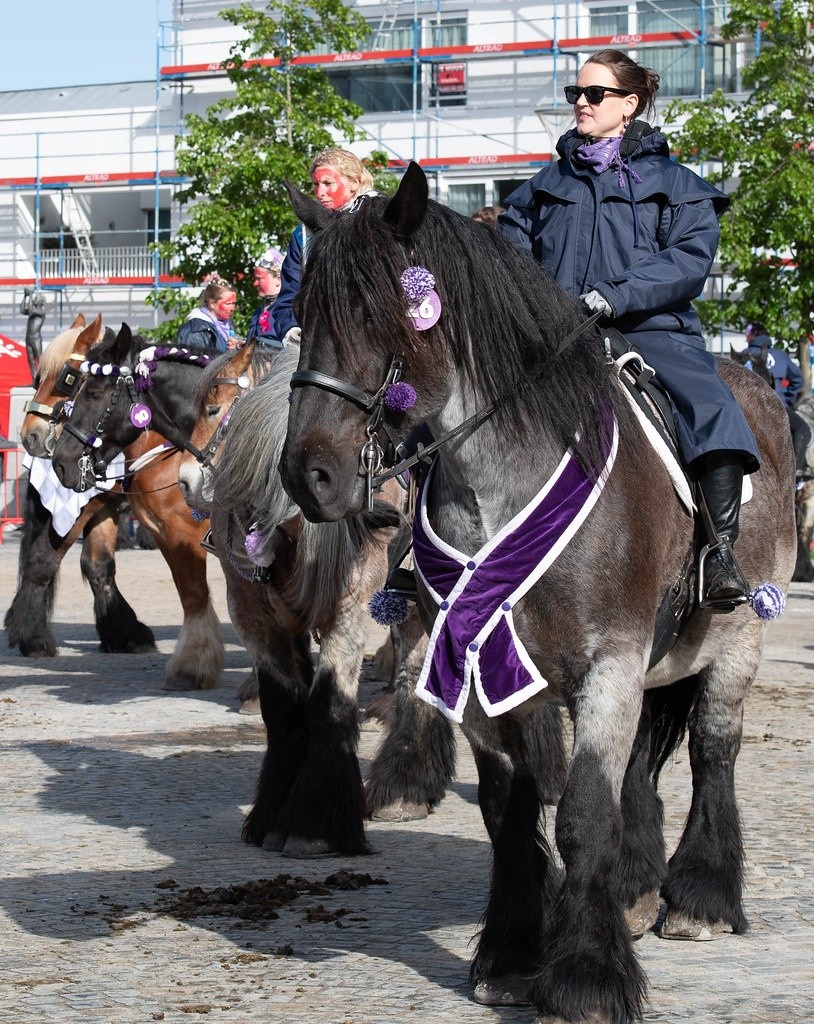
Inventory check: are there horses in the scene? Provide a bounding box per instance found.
[727,343,814,583]
[4,313,397,715]
[276,157,796,1023]
[209,336,456,857]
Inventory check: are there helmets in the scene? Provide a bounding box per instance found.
[745,323,770,341]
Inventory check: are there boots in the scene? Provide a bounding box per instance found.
[794,431,814,478]
[702,449,748,613]
[387,568,418,591]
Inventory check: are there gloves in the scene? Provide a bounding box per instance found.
[579,289,612,318]
[282,326,302,348]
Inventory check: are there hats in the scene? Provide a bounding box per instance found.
[254,246,286,272]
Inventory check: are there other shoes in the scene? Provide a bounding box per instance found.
[262,547,293,575]
[209,534,215,547]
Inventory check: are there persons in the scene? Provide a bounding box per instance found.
[175,279,241,352]
[739,323,814,483]
[498,48,761,614]
[471,206,505,227]
[246,245,286,341]
[272,149,373,349]
[20,293,47,383]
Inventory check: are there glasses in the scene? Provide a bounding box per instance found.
[564,85,633,105]
